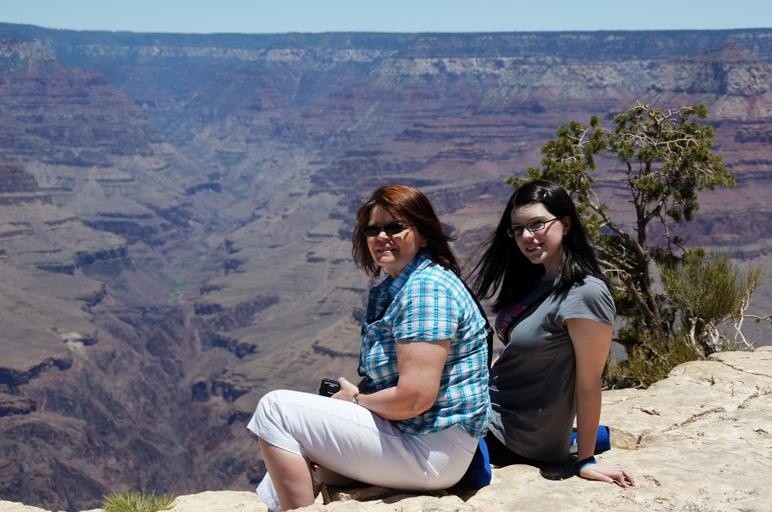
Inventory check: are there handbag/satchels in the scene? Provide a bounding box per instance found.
[570,424,610,455]
[455,436,492,488]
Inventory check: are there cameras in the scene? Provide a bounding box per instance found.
[320,379,341,397]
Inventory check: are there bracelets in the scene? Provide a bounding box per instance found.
[576,456,595,476]
[351,392,359,404]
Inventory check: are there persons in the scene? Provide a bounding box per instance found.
[464,177,638,490]
[245,184,492,511]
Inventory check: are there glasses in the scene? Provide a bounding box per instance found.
[506,217,560,238]
[363,220,417,237]
